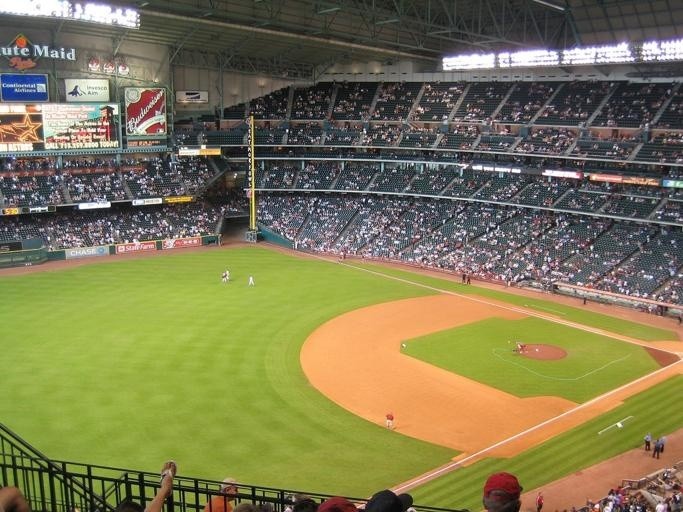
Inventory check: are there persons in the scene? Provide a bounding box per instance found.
[146,461,176,512]
[205,478,238,511]
[587,465,683,512]
[651,438,662,459]
[251,80,683,312]
[222,269,230,283]
[176,122,211,145]
[536,492,544,512]
[236,503,273,512]
[1,156,249,250]
[293,489,413,512]
[660,436,664,452]
[118,501,143,512]
[0,486,29,512]
[643,431,650,450]
[483,471,523,512]
[248,275,254,285]
[385,411,394,430]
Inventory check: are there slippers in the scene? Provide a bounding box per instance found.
[159,459,176,498]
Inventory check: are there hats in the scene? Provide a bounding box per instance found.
[365,489,413,512]
[481,472,523,501]
[220,477,242,490]
[317,496,359,511]
